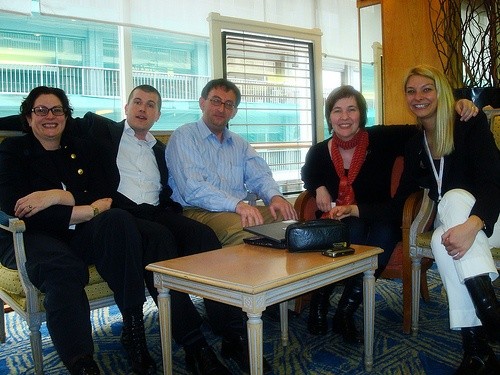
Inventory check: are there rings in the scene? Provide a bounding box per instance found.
[26,205,32,212]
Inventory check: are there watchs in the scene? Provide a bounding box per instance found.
[90,205,99,216]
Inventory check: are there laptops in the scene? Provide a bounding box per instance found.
[240,218,299,248]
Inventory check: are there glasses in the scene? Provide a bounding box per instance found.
[207,97,237,110]
[32,106,69,116]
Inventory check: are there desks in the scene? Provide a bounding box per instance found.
[146,244,385,375]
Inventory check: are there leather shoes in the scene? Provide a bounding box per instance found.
[185,338,233,375]
[222,332,274,375]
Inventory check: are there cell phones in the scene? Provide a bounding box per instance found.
[321,246,355,258]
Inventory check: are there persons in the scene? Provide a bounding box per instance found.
[165,79,299,322]
[0,84,280,375]
[301,85,478,345]
[328,65,500,375]
[0,86,157,375]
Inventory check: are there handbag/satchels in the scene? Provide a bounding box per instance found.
[285,219,351,252]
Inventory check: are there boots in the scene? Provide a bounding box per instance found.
[308,283,335,335]
[69,360,100,375]
[464,273,500,345]
[332,284,364,344]
[118,303,157,375]
[455,325,500,375]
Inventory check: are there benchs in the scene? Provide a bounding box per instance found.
[0,131,175,375]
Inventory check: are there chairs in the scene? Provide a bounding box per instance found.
[293,185,437,333]
[411,108,500,336]
